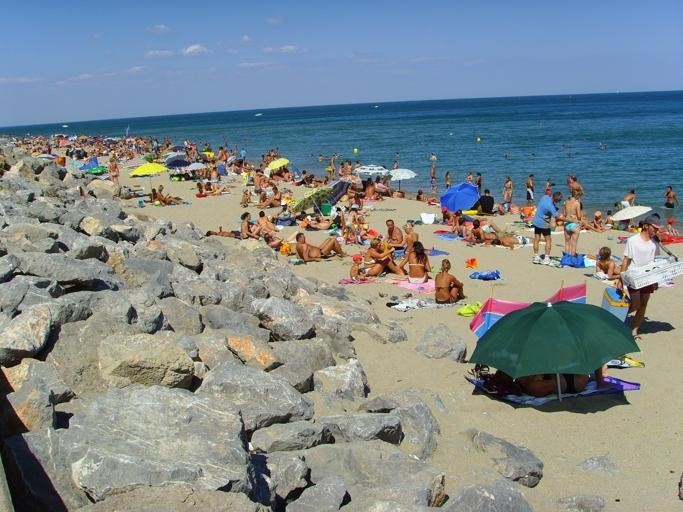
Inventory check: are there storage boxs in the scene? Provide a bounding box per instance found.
[600,286,631,323]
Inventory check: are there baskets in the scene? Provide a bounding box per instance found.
[620,256,682,290]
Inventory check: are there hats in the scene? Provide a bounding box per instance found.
[643,215,664,229]
